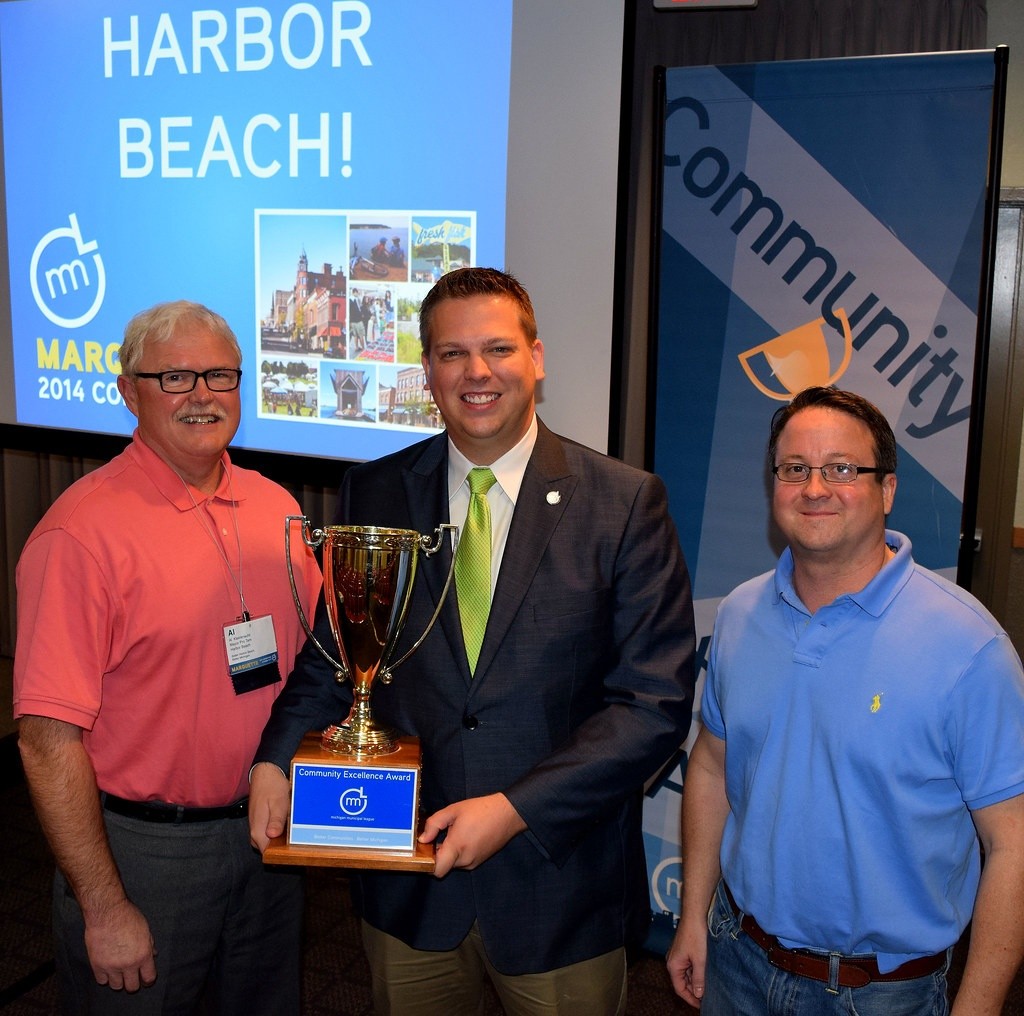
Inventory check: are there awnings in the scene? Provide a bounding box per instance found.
[392,409,420,414]
[379,409,386,413]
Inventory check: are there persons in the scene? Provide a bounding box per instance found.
[265,393,316,416]
[666,388,1024,1016]
[10,302,324,1016]
[350,287,394,352]
[249,266,695,1016]
[371,236,404,266]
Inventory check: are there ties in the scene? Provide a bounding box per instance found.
[453,467,497,677]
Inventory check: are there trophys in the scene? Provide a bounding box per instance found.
[264,516,459,872]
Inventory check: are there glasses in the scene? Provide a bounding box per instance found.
[133,367,244,395]
[772,461,893,484]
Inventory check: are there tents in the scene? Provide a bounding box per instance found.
[261,373,317,407]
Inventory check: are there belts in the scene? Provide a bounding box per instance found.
[103,792,250,825]
[723,880,946,989]
